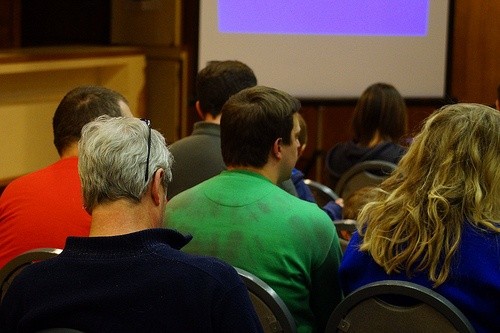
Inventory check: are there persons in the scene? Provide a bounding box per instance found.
[165,85,344,333]
[1,114,262,333]
[277,82,500,333]
[0,87,133,269]
[165,60,298,200]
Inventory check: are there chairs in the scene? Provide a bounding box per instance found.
[0,160,476,333]
